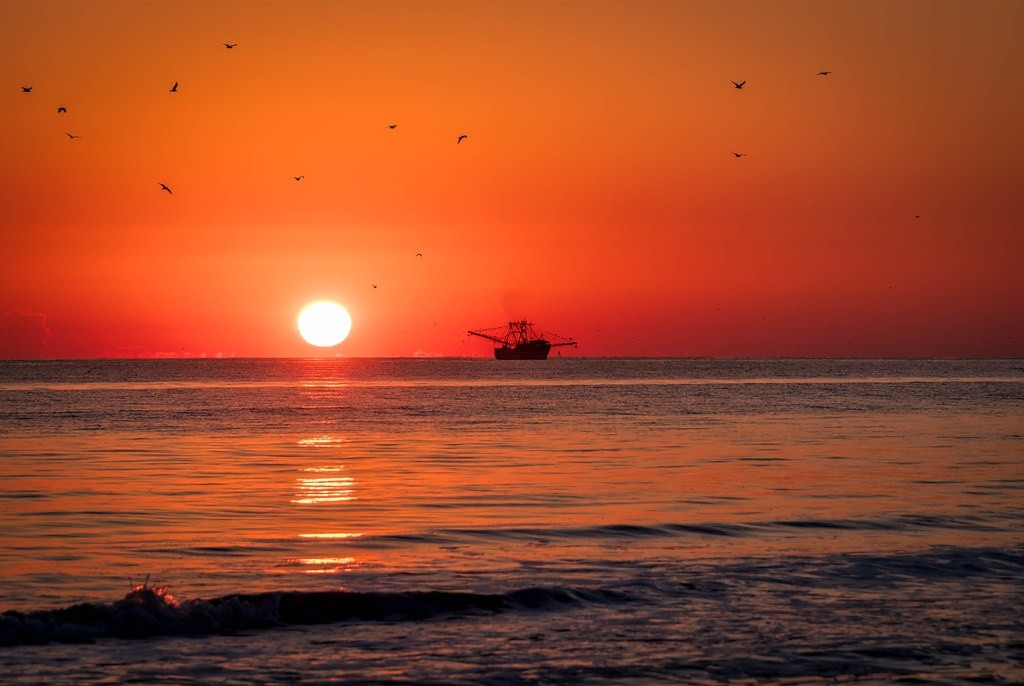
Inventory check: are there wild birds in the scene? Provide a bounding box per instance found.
[373,285,376,288]
[225,43,237,49]
[416,254,422,256]
[457,135,467,144]
[159,184,172,195]
[294,176,304,181]
[169,82,178,92]
[817,71,831,75]
[65,133,81,138]
[389,125,397,129]
[58,108,66,113]
[733,152,747,157]
[21,87,32,92]
[731,81,745,89]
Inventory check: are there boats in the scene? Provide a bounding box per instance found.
[467,317,580,361]
